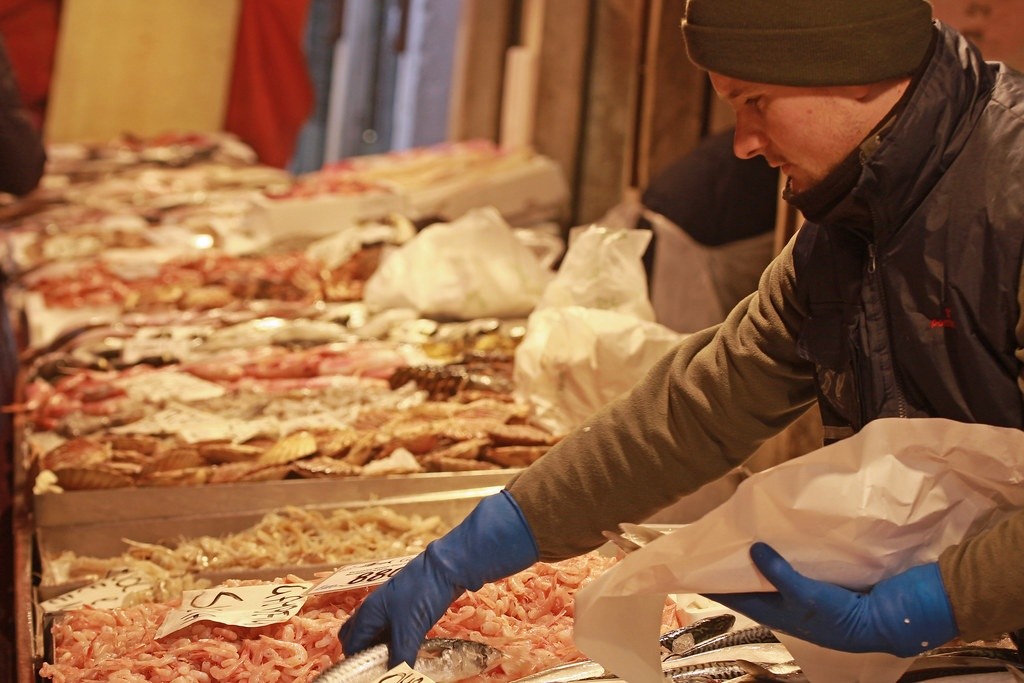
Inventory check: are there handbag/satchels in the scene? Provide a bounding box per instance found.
[366,207,564,320]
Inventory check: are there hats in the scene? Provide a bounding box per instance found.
[680,0,933,86]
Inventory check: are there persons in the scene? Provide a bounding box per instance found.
[629,125,780,333]
[337,0,1023,663]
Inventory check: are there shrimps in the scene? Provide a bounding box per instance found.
[39,507,684,682]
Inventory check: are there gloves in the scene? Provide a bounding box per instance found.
[704,543,960,658]
[337,490,540,671]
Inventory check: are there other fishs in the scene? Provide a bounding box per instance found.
[590,614,1024,683]
[0,129,571,493]
[308,637,504,683]
